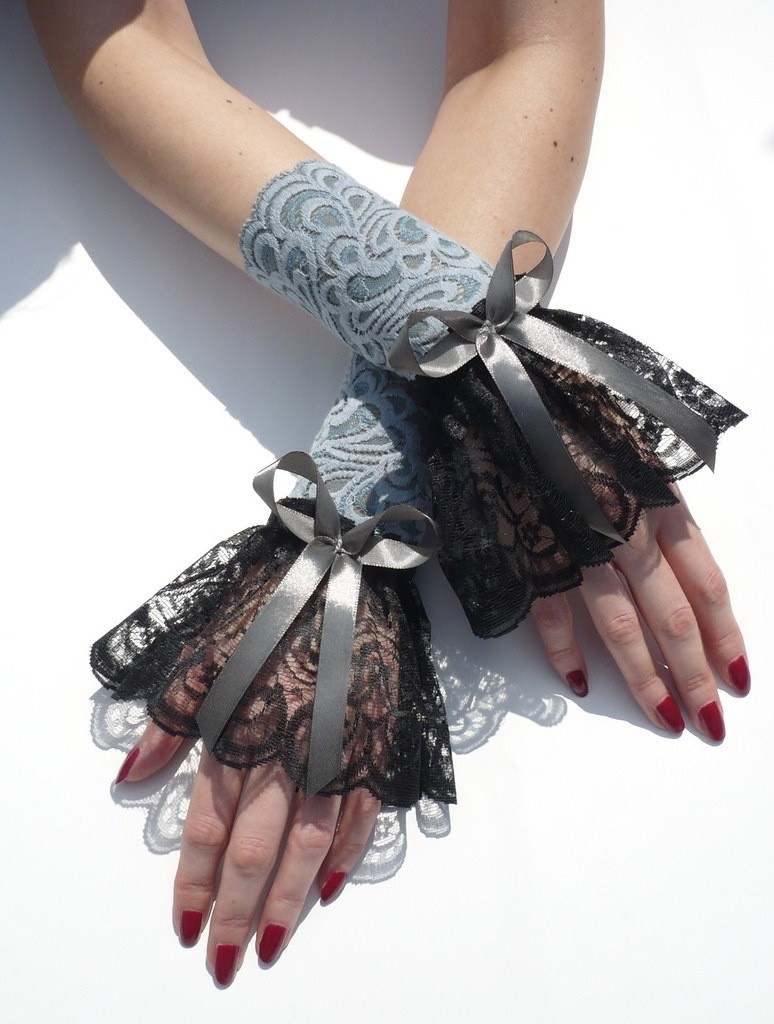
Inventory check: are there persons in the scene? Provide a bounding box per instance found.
[27,0,752,986]
